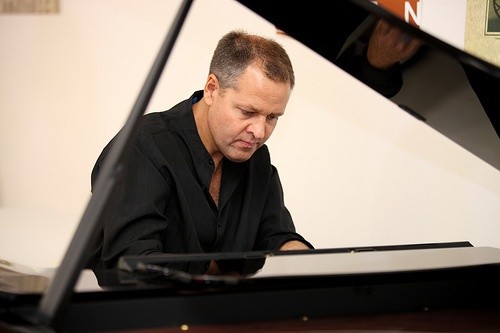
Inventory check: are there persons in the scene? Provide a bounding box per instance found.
[90,29,313,282]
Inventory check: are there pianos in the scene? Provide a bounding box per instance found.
[2,1,500,332]
